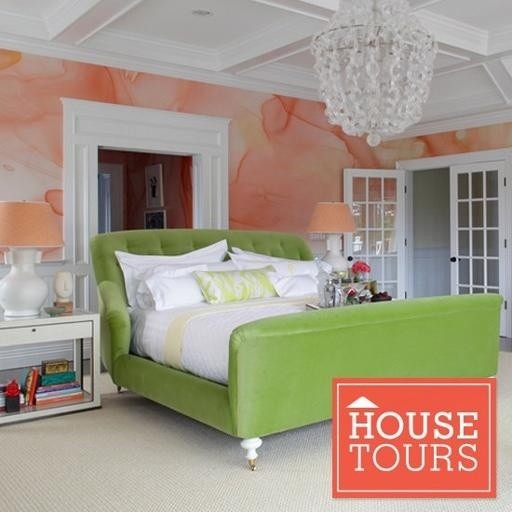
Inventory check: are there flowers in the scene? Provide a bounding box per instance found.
[351,263,371,274]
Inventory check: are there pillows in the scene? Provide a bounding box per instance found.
[112,239,331,312]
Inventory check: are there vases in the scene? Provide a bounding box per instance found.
[355,272,363,281]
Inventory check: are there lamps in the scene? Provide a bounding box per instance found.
[308,0,440,149]
[0,199,66,320]
[307,200,358,275]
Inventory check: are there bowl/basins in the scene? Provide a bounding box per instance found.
[43,307,65,318]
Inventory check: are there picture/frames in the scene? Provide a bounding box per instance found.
[144,163,165,209]
[144,210,167,230]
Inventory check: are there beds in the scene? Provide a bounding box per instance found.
[90,227,504,474]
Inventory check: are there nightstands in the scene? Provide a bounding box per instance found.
[1,309,102,425]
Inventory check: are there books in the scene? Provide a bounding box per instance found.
[0,359,85,412]
